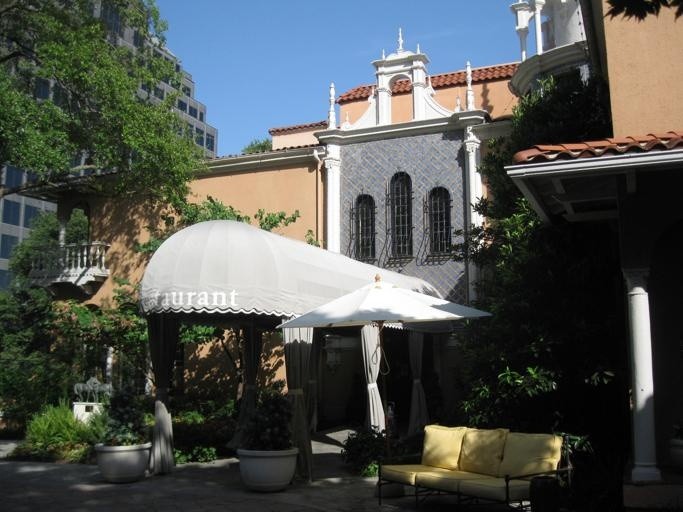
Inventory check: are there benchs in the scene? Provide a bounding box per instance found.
[377,424,574,512]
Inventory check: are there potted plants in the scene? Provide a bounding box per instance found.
[93,383,152,484]
[235,387,300,492]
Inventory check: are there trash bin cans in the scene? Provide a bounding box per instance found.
[73,401,104,424]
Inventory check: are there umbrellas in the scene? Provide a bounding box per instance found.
[274,273,494,464]
